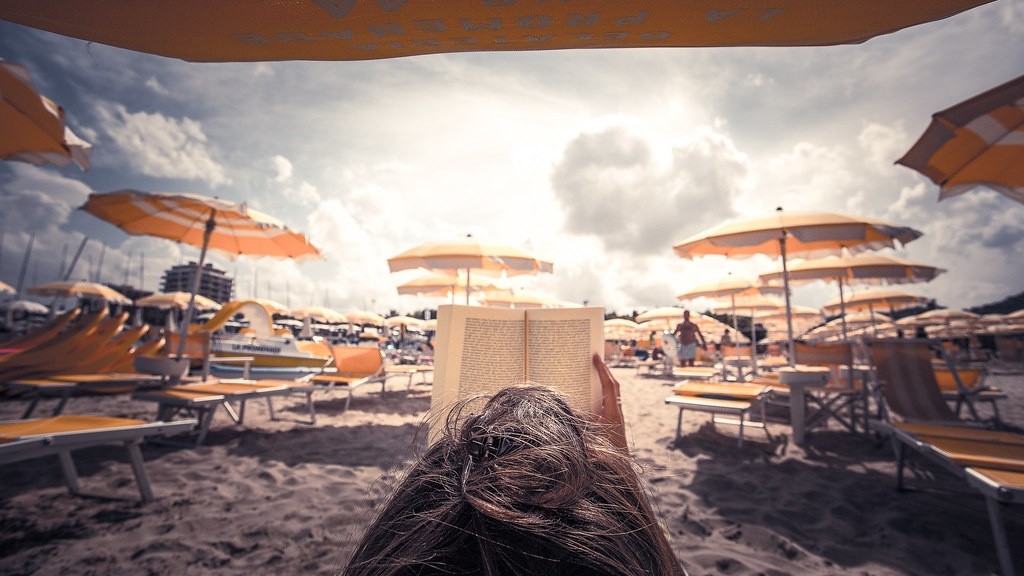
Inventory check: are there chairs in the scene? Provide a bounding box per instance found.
[0,302,1024,576]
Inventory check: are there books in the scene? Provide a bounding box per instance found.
[425,303,605,453]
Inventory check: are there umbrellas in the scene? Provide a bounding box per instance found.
[673,204,926,366]
[0,234,556,335]
[0,60,93,161]
[0,0,998,64]
[604,249,1024,347]
[79,188,326,359]
[893,75,1024,210]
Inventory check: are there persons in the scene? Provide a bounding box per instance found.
[333,353,692,576]
